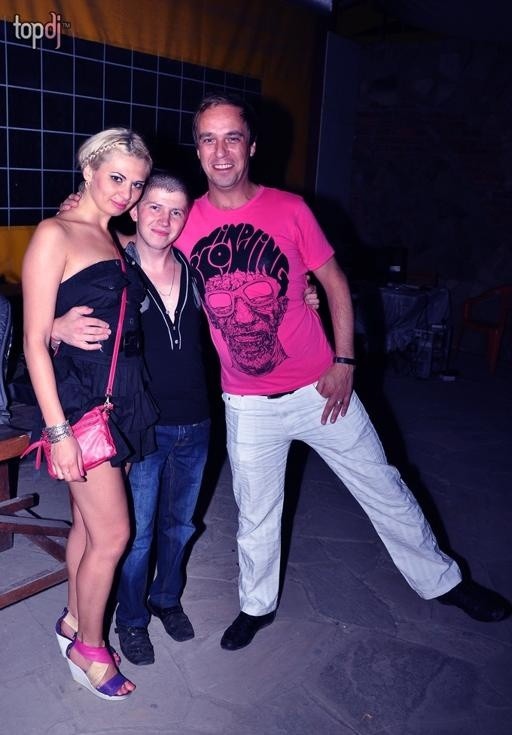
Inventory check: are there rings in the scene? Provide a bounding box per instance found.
[310,286,316,293]
[62,469,70,475]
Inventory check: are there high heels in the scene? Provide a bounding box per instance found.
[55,607,131,701]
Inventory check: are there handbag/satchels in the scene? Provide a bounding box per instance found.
[42,404,117,480]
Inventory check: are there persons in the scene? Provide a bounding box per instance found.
[17,128,163,700]
[46,171,324,663]
[53,95,512,652]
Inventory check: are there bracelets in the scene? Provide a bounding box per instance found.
[42,420,73,444]
[332,355,357,365]
[48,329,61,351]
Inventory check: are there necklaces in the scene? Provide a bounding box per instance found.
[143,259,175,298]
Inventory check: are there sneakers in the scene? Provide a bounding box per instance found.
[435,576,512,623]
[114,619,155,666]
[147,596,194,642]
[220,611,276,650]
